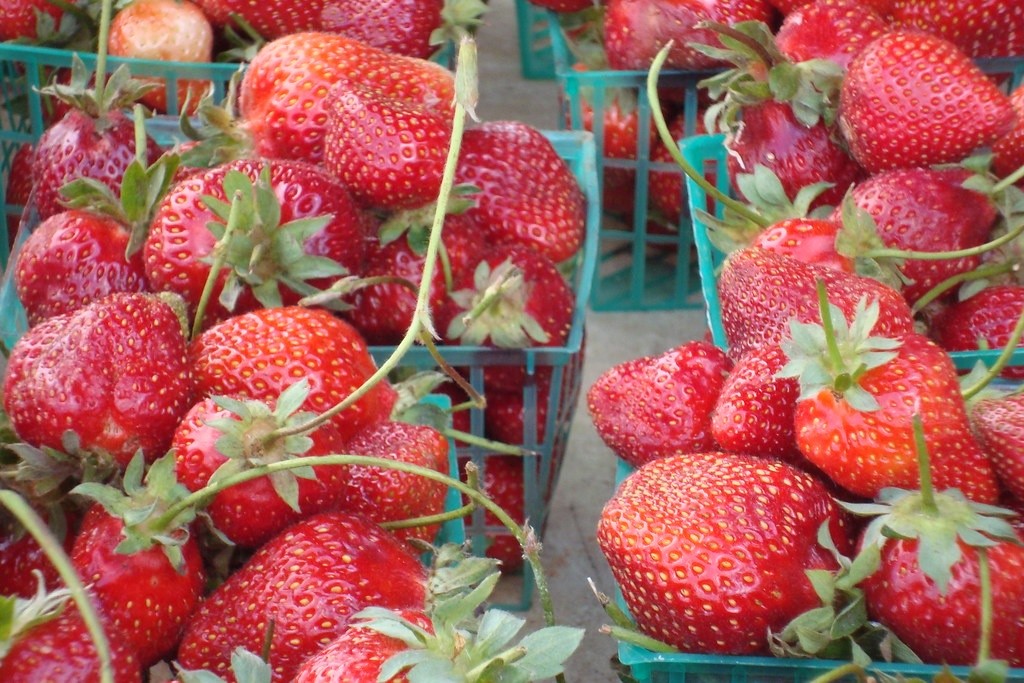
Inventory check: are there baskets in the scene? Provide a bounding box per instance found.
[614,378,1024,683]
[546,10,1024,311]
[0,0,489,268]
[0,109,601,613]
[0,393,466,683]
[516,0,606,78]
[678,134,1024,368]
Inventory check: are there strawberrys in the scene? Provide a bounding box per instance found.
[0,0,1024,682]
